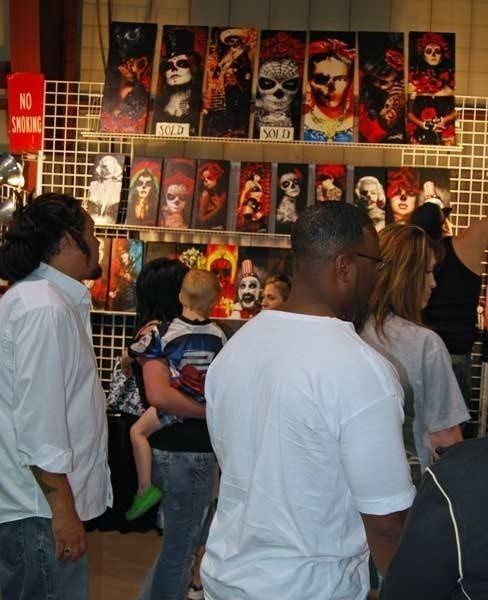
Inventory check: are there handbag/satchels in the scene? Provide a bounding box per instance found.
[106,323,166,418]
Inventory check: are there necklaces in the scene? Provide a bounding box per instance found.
[308,107,348,138]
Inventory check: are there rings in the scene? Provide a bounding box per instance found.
[63,547,71,554]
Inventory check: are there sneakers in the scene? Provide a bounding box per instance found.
[187,584,202,598]
[126,484,162,522]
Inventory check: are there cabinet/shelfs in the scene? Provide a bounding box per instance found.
[72,116,466,325]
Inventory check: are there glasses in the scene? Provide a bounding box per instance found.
[355,249,386,272]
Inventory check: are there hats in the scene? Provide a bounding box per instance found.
[117,239,128,254]
[410,202,452,235]
[423,181,439,200]
[240,260,258,278]
[129,162,159,192]
[221,29,249,47]
[318,165,344,178]
[164,159,193,193]
[115,32,149,65]
[164,29,198,56]
[256,167,264,176]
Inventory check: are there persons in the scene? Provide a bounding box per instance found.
[238,169,266,225]
[354,175,387,232]
[88,154,122,224]
[407,33,456,144]
[304,40,356,142]
[255,34,302,121]
[357,221,471,488]
[129,256,218,600]
[275,168,301,224]
[159,171,195,231]
[198,198,417,600]
[108,247,138,311]
[119,268,228,522]
[261,273,291,310]
[408,195,488,391]
[128,168,159,226]
[1,192,115,600]
[151,27,201,136]
[387,167,420,225]
[198,161,227,226]
[235,267,267,316]
[218,28,253,133]
